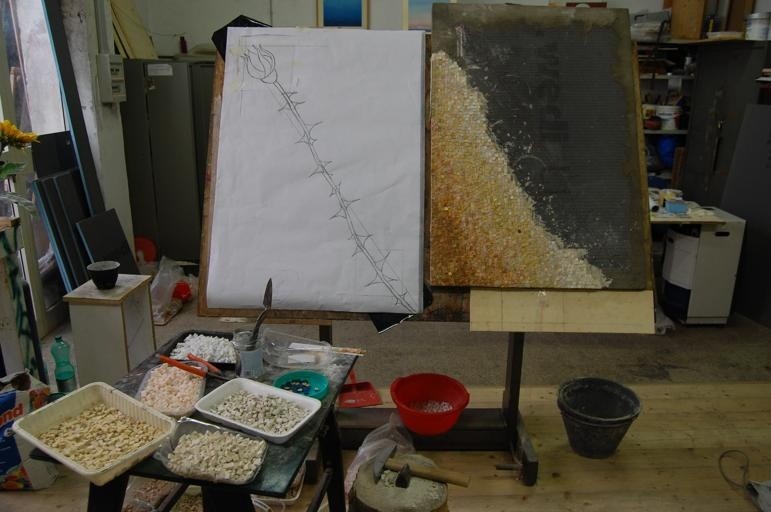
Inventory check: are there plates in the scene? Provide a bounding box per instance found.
[197,377,322,443]
[163,328,241,370]
[13,382,174,486]
[154,416,268,483]
[133,362,209,417]
[272,369,329,399]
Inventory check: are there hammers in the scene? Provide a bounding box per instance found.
[371,443,470,491]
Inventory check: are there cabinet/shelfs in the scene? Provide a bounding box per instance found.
[120,54,220,275]
[658,202,746,331]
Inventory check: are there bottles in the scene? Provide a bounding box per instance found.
[50,335,77,394]
[745,11,770,41]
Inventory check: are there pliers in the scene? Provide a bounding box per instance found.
[151,352,227,382]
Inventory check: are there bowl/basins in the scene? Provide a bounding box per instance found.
[559,377,642,459]
[88,260,120,290]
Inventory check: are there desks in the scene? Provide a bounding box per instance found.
[85,329,362,511]
[61,273,155,386]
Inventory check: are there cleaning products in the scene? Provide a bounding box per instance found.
[51,335,75,380]
[0,389,60,491]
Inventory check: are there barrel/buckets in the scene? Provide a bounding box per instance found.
[556,377,642,458]
